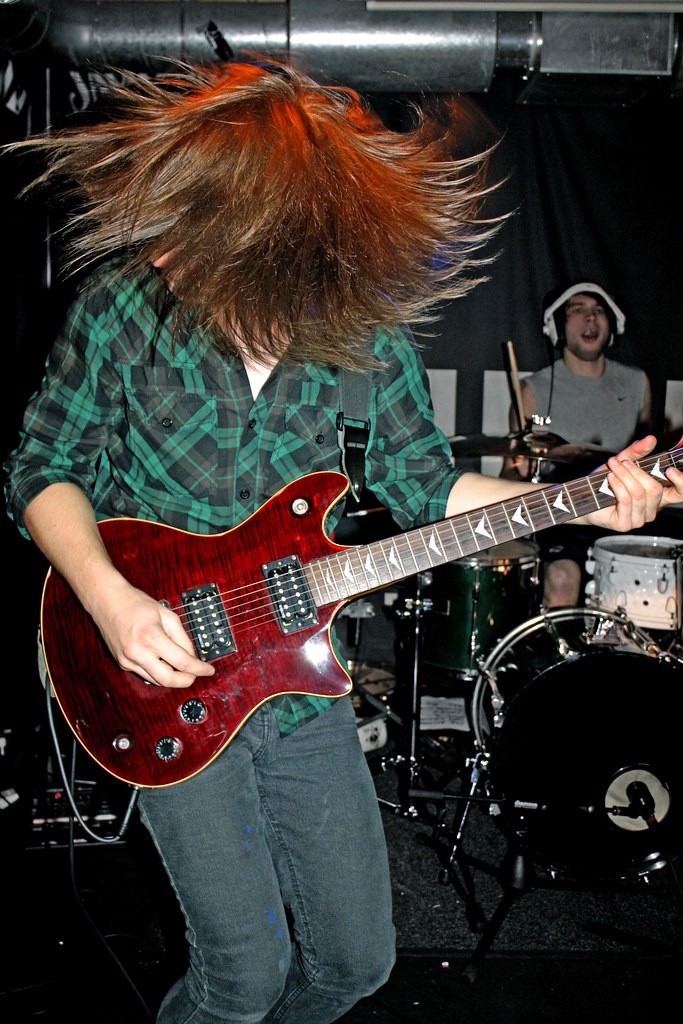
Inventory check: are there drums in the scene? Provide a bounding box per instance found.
[590,534,682,631]
[419,538,542,675]
[470,605,683,888]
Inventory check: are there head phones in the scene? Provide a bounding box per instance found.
[542,313,616,351]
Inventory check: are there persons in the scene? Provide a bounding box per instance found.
[2,49,683,1024]
[508,279,651,610]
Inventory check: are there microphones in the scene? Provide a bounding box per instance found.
[627,780,657,829]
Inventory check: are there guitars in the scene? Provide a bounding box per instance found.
[32,443,683,793]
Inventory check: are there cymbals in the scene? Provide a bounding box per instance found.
[453,415,573,465]
